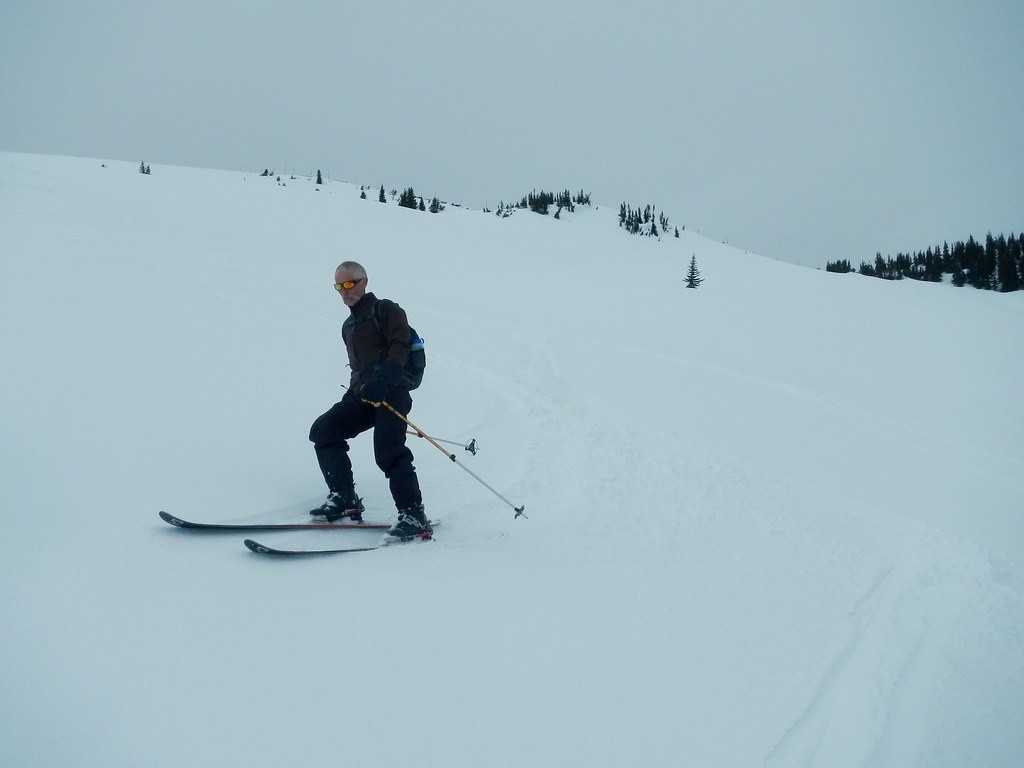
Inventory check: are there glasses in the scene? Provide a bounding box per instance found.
[334,279,361,291]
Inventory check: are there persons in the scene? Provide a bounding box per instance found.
[308,262,433,538]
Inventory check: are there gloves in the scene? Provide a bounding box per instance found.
[359,377,389,407]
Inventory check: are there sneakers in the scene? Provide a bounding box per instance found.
[383,503,433,541]
[308,491,366,524]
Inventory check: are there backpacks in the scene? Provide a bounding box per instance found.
[372,298,426,392]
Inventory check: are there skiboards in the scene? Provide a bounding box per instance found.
[158,510,442,558]
